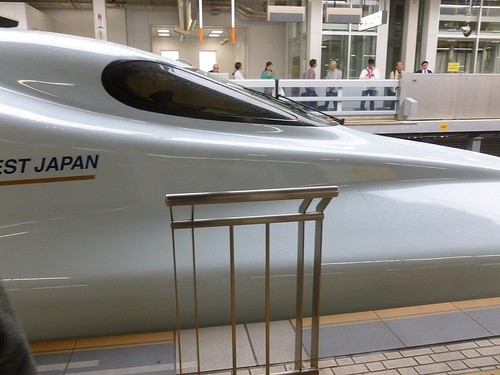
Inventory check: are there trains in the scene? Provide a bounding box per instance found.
[0,27,500,343]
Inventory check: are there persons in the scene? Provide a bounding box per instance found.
[415,61,433,73]
[232,62,245,81]
[324,60,342,110]
[359,59,380,110]
[388,62,405,110]
[305,59,319,110]
[208,64,220,73]
[261,62,278,96]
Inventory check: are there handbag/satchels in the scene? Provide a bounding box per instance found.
[387,88,396,96]
